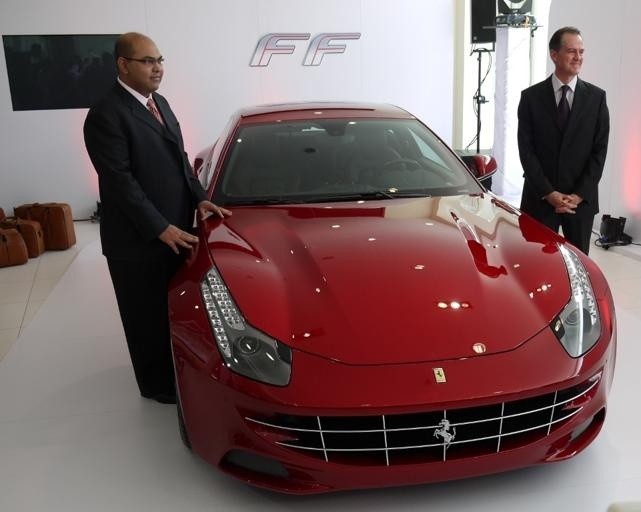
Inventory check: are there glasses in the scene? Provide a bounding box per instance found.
[124,57,163,65]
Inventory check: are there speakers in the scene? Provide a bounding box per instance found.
[471,1,496,43]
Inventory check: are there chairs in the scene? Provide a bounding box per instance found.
[230,134,417,196]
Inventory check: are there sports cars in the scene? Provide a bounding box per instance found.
[168,98,612,496]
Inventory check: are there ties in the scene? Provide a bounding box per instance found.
[557,85,570,117]
[146,99,160,120]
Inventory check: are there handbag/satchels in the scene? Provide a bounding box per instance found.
[0,202,75,268]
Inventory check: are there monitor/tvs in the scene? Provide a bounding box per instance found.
[3,34,123,111]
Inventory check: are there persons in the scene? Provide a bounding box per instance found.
[516,26,610,259]
[515,209,570,255]
[82,32,234,405]
